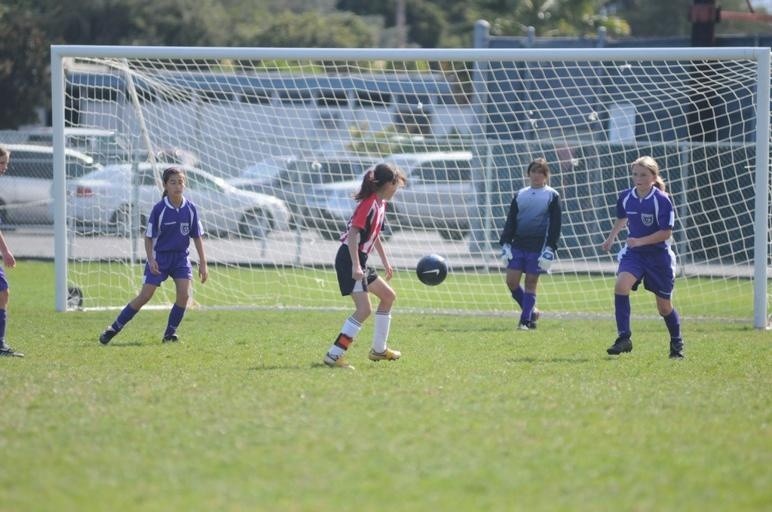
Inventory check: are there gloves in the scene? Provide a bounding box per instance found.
[537,248,555,271]
[498,243,513,268]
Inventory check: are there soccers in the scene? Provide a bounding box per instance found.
[416,254,447,285]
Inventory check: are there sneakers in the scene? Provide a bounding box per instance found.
[162,335,179,343]
[368,347,401,360]
[668,340,685,359]
[0,347,24,357]
[516,308,540,330]
[100,325,118,344]
[323,351,355,369]
[606,336,633,354]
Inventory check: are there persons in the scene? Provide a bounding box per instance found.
[99,167,208,344]
[322,162,407,366]
[499,157,563,331]
[0,146,24,358]
[600,154,686,359]
